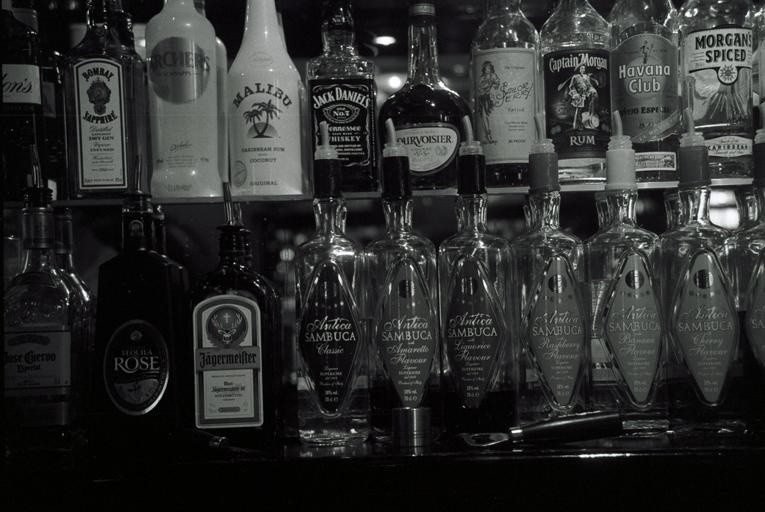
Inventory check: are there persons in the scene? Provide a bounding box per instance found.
[478,61,501,142]
[569,65,600,132]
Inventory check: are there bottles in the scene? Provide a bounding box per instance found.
[1,105,764,447]
[0,1,765,204]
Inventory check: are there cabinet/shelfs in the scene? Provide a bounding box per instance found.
[0,175,765,490]
